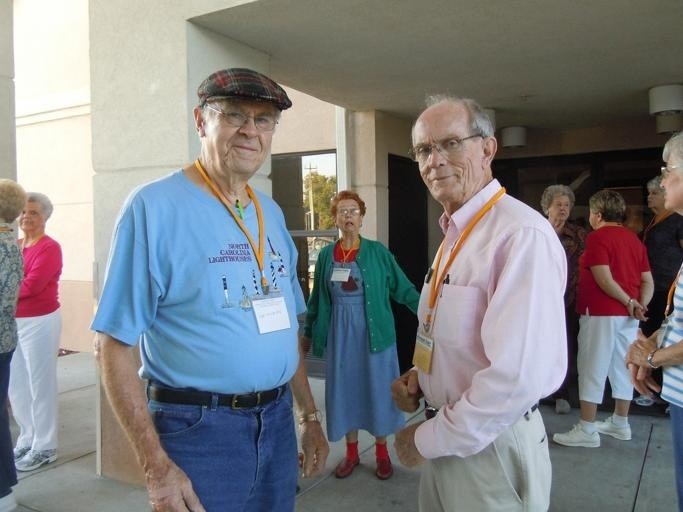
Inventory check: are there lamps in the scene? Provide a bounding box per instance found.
[501,126,527,147]
[647,84,683,135]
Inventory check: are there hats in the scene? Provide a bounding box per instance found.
[197,68,292,109]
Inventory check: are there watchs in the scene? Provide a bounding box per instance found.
[298,410,322,424]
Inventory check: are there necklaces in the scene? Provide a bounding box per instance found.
[22,235,42,250]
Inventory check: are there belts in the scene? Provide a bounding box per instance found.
[147,383,289,410]
[424,400,539,420]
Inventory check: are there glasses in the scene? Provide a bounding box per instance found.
[340,208,361,217]
[407,134,484,162]
[660,166,680,178]
[204,104,279,132]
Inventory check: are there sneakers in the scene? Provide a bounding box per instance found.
[596,416,632,440]
[335,455,360,478]
[13,446,57,472]
[0,483,22,512]
[376,456,393,480]
[555,399,571,415]
[634,395,668,406]
[552,424,600,448]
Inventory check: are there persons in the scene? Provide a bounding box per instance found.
[0,178,24,512]
[91,69,329,512]
[391,95,569,512]
[540,131,683,511]
[301,191,420,479]
[8,193,62,472]
